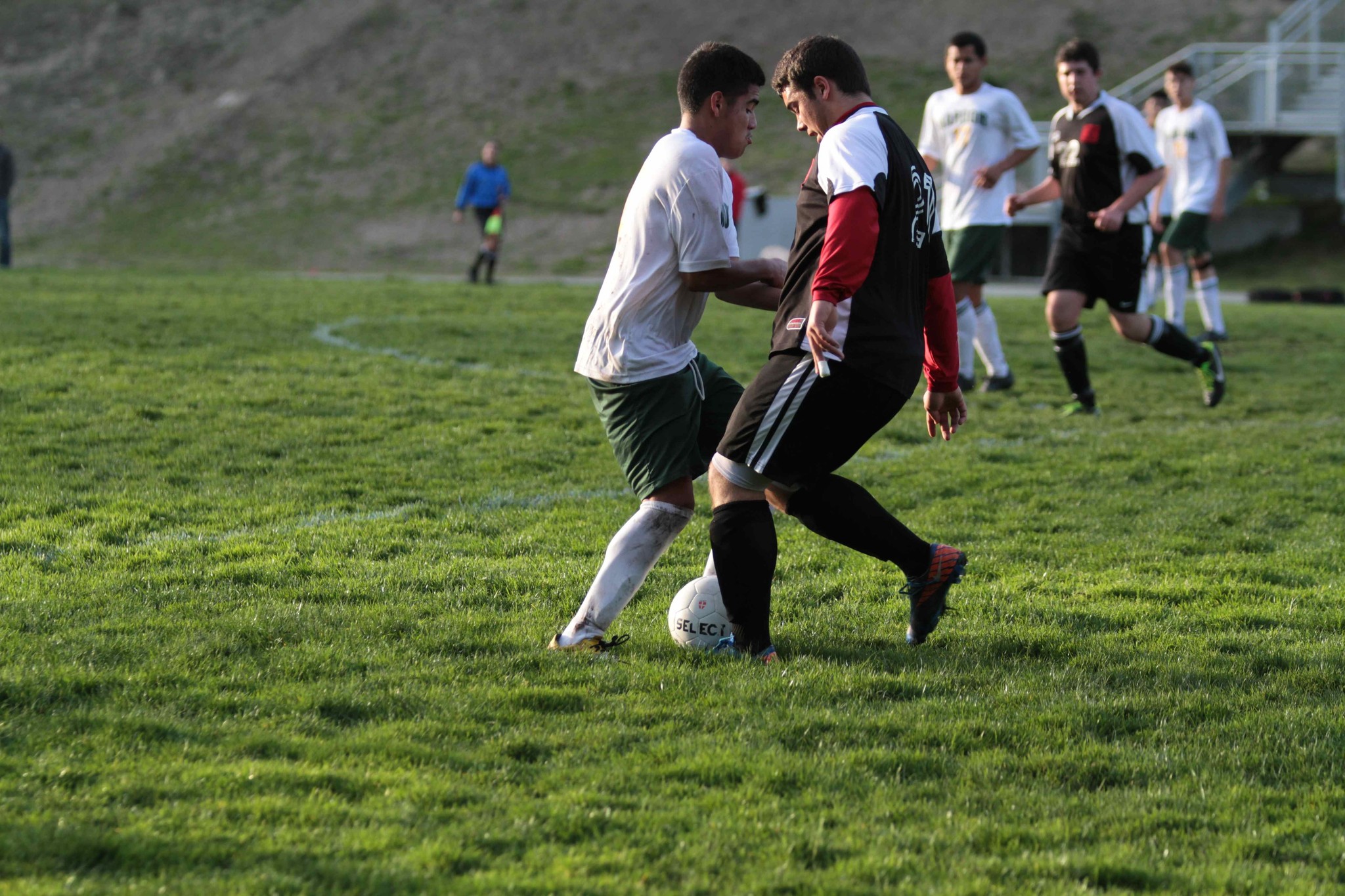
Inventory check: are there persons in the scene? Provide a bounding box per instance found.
[1003,36,1227,421]
[0,144,17,268]
[915,32,1041,398]
[454,138,513,287]
[707,33,970,665]
[545,39,784,656]
[720,154,746,247]
[1155,61,1234,346]
[1139,86,1173,319]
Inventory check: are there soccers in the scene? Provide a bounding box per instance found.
[668,575,733,653]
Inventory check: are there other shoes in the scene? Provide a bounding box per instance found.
[1192,330,1230,342]
[468,266,476,282]
[484,279,496,286]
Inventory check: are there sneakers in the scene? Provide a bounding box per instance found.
[907,542,969,645]
[548,632,610,654]
[1195,341,1225,408]
[980,369,1016,391]
[957,372,975,393]
[703,642,781,663]
[1059,402,1101,415]
